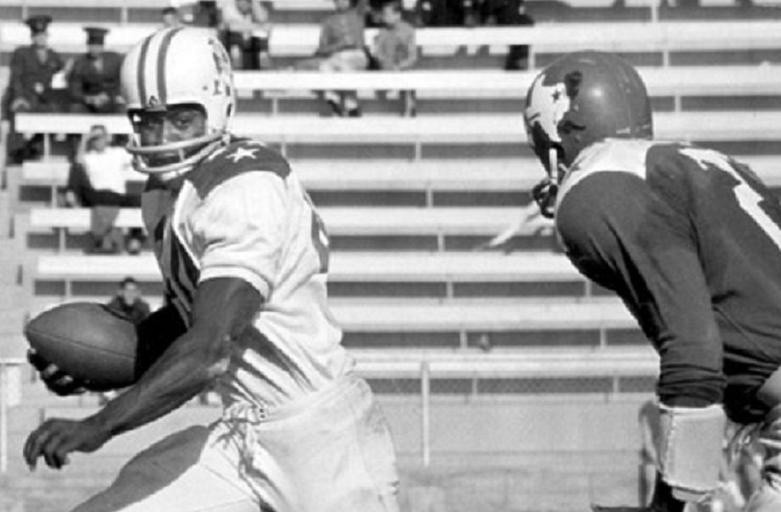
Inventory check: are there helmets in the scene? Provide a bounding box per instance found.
[120,27,235,184]
[525,52,652,217]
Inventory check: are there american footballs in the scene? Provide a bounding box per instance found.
[24,304,142,388]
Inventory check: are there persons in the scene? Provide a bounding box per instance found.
[108,277,151,321]
[319,0,417,117]
[471,0,532,70]
[195,0,270,71]
[19,21,401,512]
[1,14,145,254]
[524,51,780,511]
